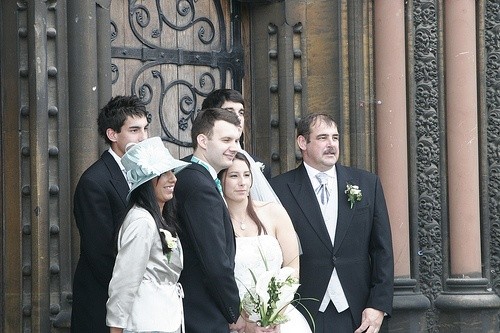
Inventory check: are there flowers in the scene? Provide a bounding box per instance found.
[164,238,177,264]
[234,263,320,331]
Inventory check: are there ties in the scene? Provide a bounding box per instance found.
[316,172,329,206]
[190,156,224,194]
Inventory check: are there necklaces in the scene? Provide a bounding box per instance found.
[231,211,247,230]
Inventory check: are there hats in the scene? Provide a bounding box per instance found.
[120,136,192,202]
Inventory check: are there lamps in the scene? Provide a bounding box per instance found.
[345,184,363,210]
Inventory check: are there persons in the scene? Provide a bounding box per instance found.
[70,94,149,333]
[106,137,191,333]
[175,108,246,333]
[268,111,395,333]
[181,88,246,162]
[218,148,313,333]
